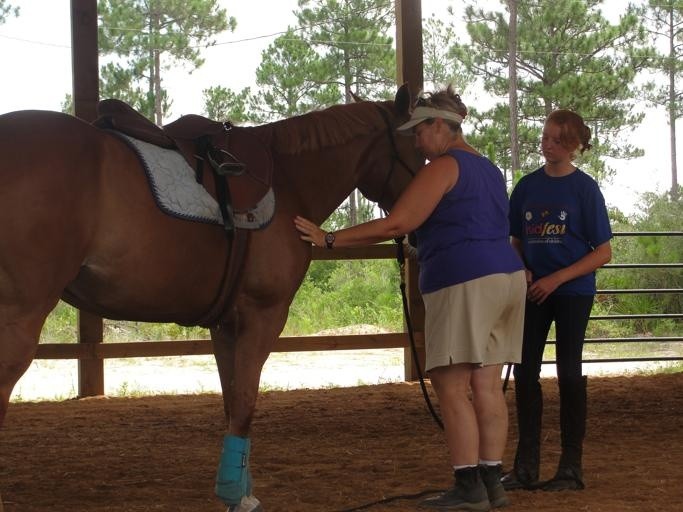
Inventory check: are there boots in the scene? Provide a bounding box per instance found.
[539,374,589,494]
[501,378,543,488]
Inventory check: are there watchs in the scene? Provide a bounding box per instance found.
[326,229,335,250]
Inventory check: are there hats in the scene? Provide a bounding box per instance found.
[396,106,465,138]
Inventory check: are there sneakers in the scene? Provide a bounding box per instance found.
[480,463,508,507]
[422,464,490,509]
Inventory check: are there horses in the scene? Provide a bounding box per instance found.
[0,82,431,512]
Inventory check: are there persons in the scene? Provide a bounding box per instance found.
[501,107,614,490]
[294,81,527,512]
